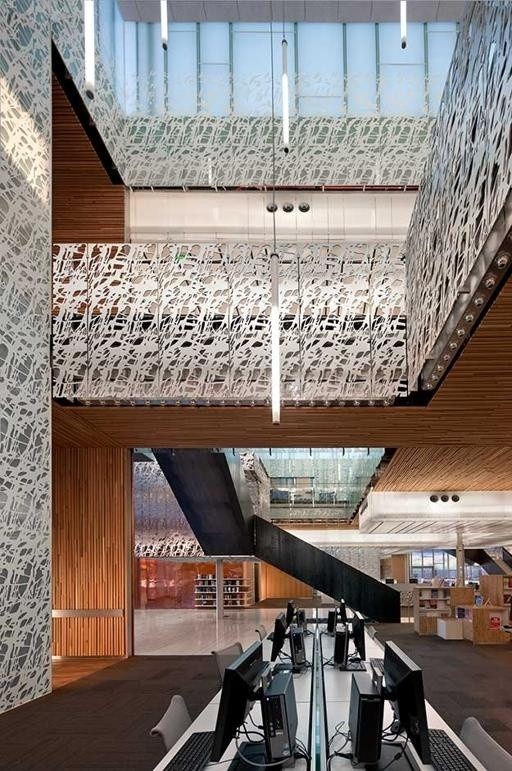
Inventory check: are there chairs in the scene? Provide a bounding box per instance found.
[457,716,512,771]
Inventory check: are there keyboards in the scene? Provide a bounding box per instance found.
[428,729,478,771]
[163,732,213,771]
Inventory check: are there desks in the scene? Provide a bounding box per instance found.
[148,607,486,771]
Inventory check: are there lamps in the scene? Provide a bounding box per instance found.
[160,2,167,48]
[83,2,95,99]
[400,0,408,51]
[281,40,293,155]
[269,251,281,425]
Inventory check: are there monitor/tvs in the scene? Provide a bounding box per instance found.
[211,598,430,771]
[410,579,417,583]
[386,579,394,584]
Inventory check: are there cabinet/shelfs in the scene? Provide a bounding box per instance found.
[194,579,251,607]
[413,586,452,636]
